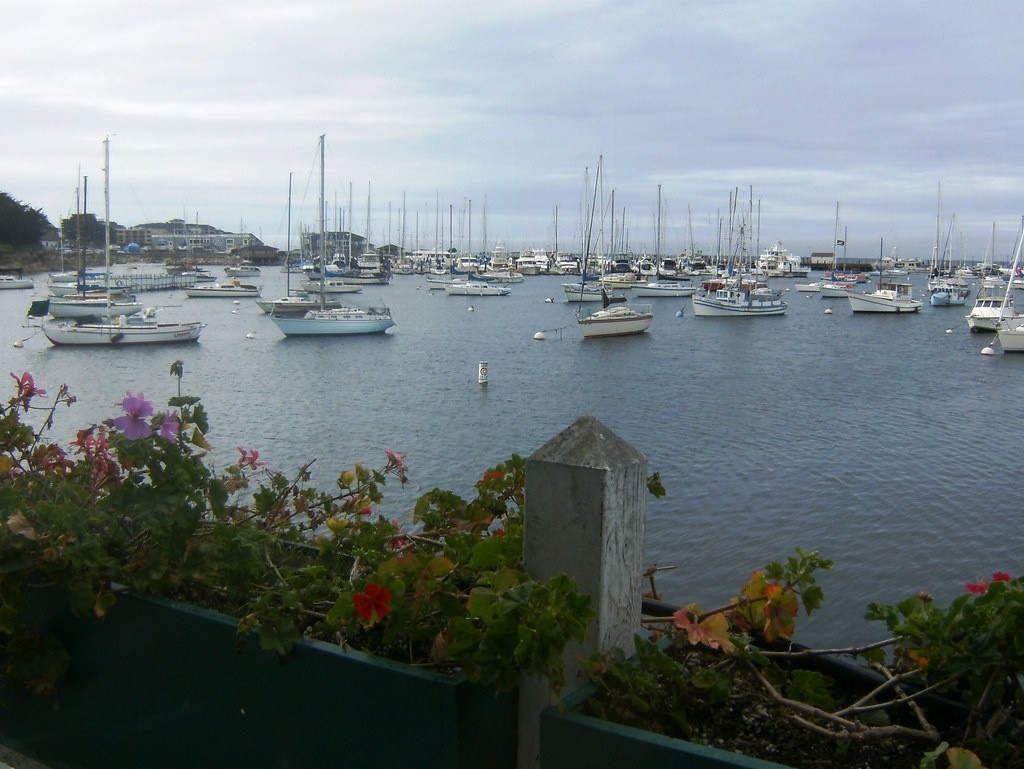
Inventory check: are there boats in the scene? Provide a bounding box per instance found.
[930,287,964,306]
[846,282,924,313]
[750,242,811,277]
[870,256,955,277]
[629,282,697,297]
[818,284,853,297]
[47,271,79,281]
[425,277,446,289]
[927,276,953,290]
[486,240,513,272]
[599,277,647,288]
[980,275,1007,288]
[470,273,525,283]
[446,283,511,296]
[794,281,820,291]
[699,276,767,290]
[562,283,613,301]
[821,265,871,283]
[1008,278,1024,290]
[167,264,201,274]
[183,273,217,282]
[183,282,263,297]
[302,280,363,292]
[575,306,654,335]
[964,298,1016,332]
[515,249,541,275]
[48,284,79,296]
[992,317,1024,352]
[226,266,263,276]
[689,291,788,316]
[0,275,34,289]
[255,297,343,313]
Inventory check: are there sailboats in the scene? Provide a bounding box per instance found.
[41,138,207,343]
[272,134,394,335]
[392,187,480,274]
[550,152,659,276]
[283,170,388,285]
[45,176,144,318]
[955,216,1024,278]
[681,185,754,277]
